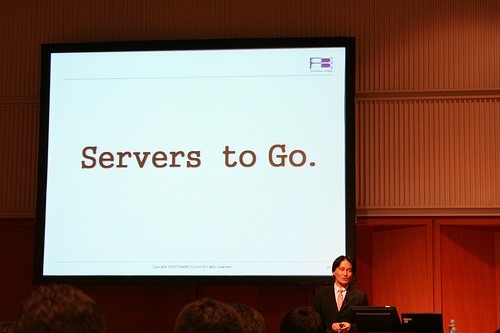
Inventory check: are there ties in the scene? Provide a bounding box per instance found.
[336,289,343,311]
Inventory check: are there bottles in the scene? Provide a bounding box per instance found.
[449,319,457,333]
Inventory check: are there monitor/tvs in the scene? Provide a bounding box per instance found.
[402,313,443,333]
[348,306,401,333]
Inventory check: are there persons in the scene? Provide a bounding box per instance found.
[176,301,265,333]
[280,307,324,333]
[0,322,14,333]
[312,256,368,333]
[14,285,100,333]
